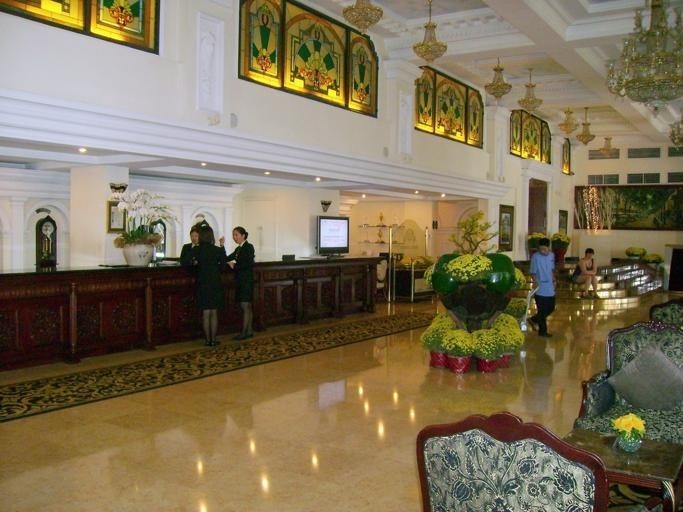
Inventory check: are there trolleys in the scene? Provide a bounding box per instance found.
[519,349,534,394]
[518,284,539,333]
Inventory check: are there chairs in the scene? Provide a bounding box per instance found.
[416,410,674,512]
[648,297,682,324]
[573,320,683,445]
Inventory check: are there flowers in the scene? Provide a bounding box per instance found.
[111,188,181,248]
[625,246,647,258]
[642,253,665,264]
[419,211,538,360]
[609,412,648,442]
[527,232,547,249]
[550,233,570,249]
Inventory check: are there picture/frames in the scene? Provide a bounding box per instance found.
[574,185,683,231]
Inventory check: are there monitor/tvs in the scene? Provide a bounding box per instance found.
[317,216,349,258]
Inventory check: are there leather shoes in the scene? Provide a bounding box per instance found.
[527,317,552,338]
[206,334,253,346]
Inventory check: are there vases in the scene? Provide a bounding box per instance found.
[552,248,567,267]
[122,242,154,269]
[616,430,643,453]
[527,247,539,262]
[630,256,640,261]
[647,263,658,270]
[428,349,513,374]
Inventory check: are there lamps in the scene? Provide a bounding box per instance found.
[483,57,513,107]
[577,106,597,150]
[560,108,577,136]
[604,1,683,115]
[413,0,447,72]
[341,0,386,38]
[665,117,683,157]
[517,68,543,117]
[596,136,614,158]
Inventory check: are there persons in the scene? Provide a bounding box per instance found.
[527,238,558,337]
[180,225,228,346]
[571,248,601,300]
[219,226,256,339]
[181,228,199,260]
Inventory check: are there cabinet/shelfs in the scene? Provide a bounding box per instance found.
[385,264,440,304]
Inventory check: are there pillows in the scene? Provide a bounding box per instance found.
[606,344,683,412]
